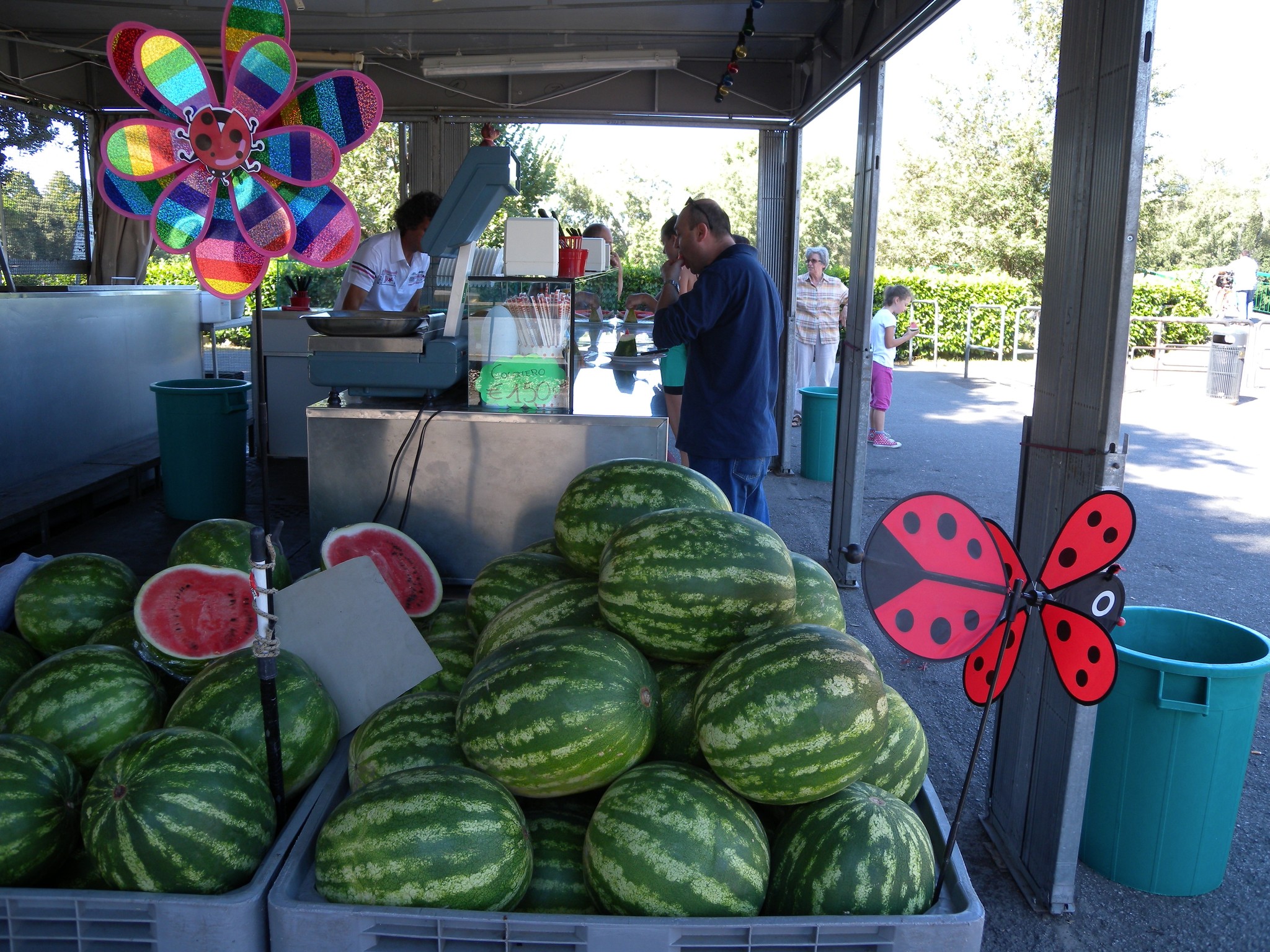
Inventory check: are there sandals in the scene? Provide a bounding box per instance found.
[792,415,802,427]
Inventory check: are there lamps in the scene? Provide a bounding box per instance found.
[420,49,679,79]
[191,47,364,71]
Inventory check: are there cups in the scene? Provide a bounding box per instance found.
[557,248,589,278]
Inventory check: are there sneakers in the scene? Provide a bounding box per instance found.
[867,428,895,442]
[872,431,902,448]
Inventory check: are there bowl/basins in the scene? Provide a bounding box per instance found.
[289,297,311,307]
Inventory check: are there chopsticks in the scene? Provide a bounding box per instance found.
[505,289,571,345]
[284,274,312,291]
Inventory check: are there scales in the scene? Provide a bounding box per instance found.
[299,145,521,412]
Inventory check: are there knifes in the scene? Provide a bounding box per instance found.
[637,348,670,355]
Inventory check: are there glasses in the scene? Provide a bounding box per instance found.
[685,196,712,230]
[806,258,821,264]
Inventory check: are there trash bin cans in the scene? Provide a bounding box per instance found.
[1205,330,1248,406]
[1079,605,1270,896]
[149,377,253,521]
[798,387,838,483]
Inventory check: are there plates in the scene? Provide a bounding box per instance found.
[601,352,668,362]
[574,319,610,326]
[574,350,598,361]
[617,320,654,327]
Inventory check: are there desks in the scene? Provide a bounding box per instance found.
[200,316,252,378]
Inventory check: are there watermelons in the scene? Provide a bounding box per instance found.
[614,328,637,357]
[0,519,443,895]
[314,458,937,917]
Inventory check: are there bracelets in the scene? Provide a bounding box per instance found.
[663,280,680,296]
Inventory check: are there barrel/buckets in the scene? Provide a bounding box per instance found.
[798,385,839,481]
[149,378,253,522]
[1076,604,1270,895]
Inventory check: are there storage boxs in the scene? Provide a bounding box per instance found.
[0,743,985,952]
[504,217,610,277]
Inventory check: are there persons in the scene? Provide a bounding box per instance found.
[1229,249,1260,324]
[626,214,698,468]
[333,190,443,312]
[528,283,602,309]
[791,247,850,428]
[583,222,623,303]
[869,285,920,447]
[653,196,785,528]
